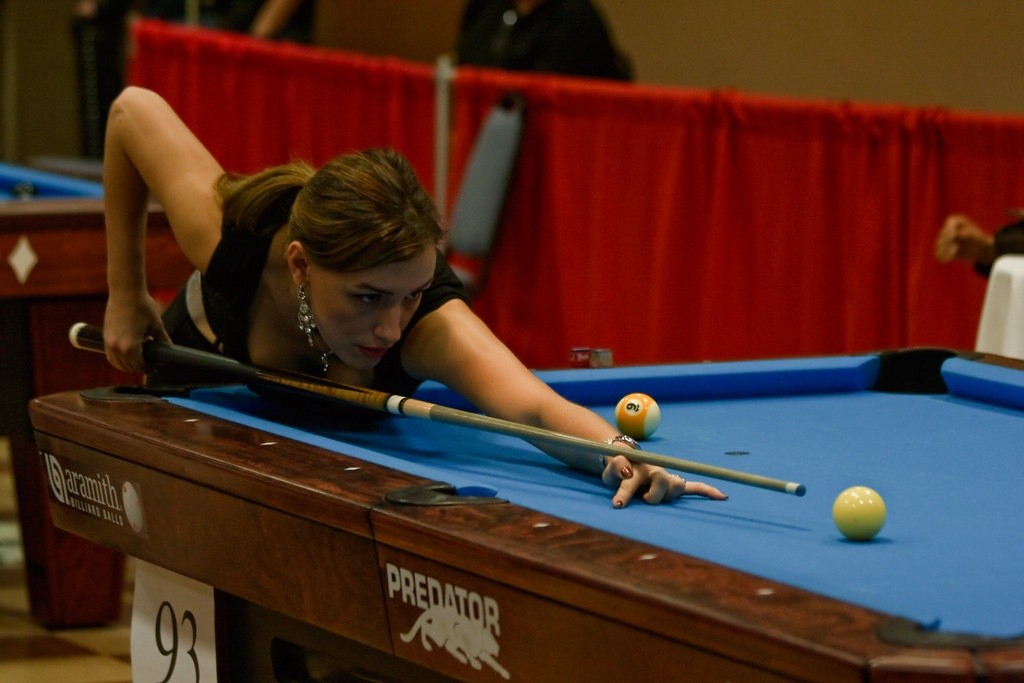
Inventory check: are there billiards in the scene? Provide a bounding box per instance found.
[829,483,887,542]
[613,391,662,440]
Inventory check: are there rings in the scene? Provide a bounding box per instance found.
[667,474,686,483]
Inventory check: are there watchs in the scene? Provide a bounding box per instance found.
[599,434,641,469]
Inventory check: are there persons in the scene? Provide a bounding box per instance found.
[76,0,315,162]
[456,0,635,83]
[103,86,728,509]
[937,213,1024,279]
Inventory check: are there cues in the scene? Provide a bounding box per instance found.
[64,319,810,500]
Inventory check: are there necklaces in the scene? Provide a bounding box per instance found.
[312,330,333,371]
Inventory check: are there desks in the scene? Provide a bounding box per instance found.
[30,348,1024,683]
[1,161,199,628]
